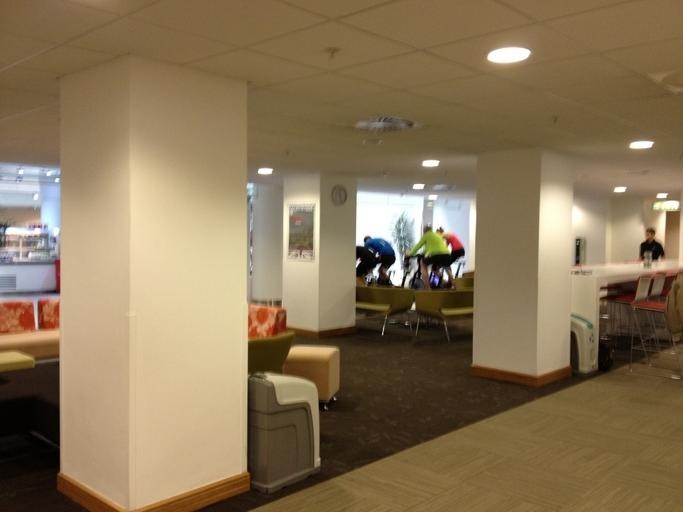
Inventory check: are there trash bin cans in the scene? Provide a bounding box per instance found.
[571,314,597,375]
[247,372,321,494]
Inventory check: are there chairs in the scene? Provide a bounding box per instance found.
[599,269,683,371]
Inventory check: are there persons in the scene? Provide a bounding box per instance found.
[356,245,377,285]
[406,223,450,289]
[436,227,465,286]
[640,228,665,260]
[364,235,396,286]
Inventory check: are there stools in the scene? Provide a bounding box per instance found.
[283,344,340,409]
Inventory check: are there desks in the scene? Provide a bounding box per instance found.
[571,263,683,371]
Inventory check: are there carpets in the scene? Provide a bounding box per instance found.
[0,312,683,511]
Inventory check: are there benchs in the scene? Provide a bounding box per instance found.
[356,273,474,341]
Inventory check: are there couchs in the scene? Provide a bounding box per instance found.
[0,297,295,403]
